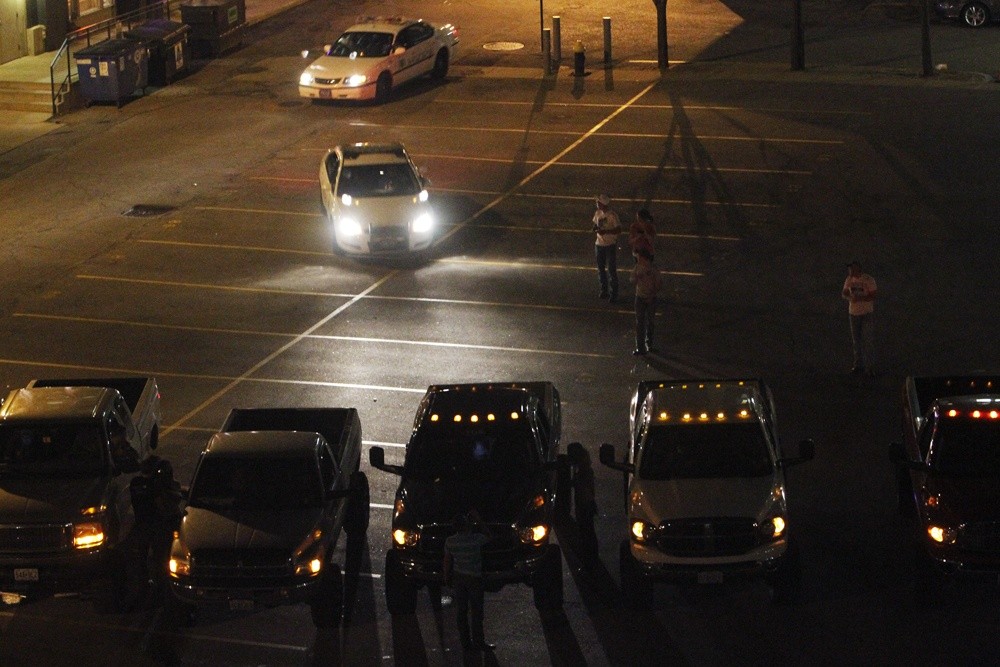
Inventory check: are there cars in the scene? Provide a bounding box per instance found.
[319,142,436,265]
[935,0,1000,28]
[298,16,460,106]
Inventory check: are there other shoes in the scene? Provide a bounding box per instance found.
[633,347,647,355]
[610,294,618,305]
[863,363,880,373]
[645,340,656,352]
[599,290,608,298]
[852,359,862,371]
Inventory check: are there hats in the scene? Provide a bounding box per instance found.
[845,260,862,272]
[595,194,610,207]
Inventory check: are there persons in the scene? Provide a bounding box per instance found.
[572,448,599,576]
[131,459,167,585]
[628,207,656,307]
[443,509,496,651]
[842,259,877,377]
[630,247,665,356]
[592,192,622,303]
[377,166,410,193]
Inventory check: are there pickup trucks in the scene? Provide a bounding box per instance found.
[369,380,572,621]
[161,406,370,630]
[598,376,817,602]
[902,366,1000,591]
[0,375,162,619]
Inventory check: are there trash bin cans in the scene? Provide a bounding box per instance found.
[179,0,249,57]
[74,38,152,108]
[122,18,192,81]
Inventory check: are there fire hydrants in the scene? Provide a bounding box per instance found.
[571,39,587,76]
